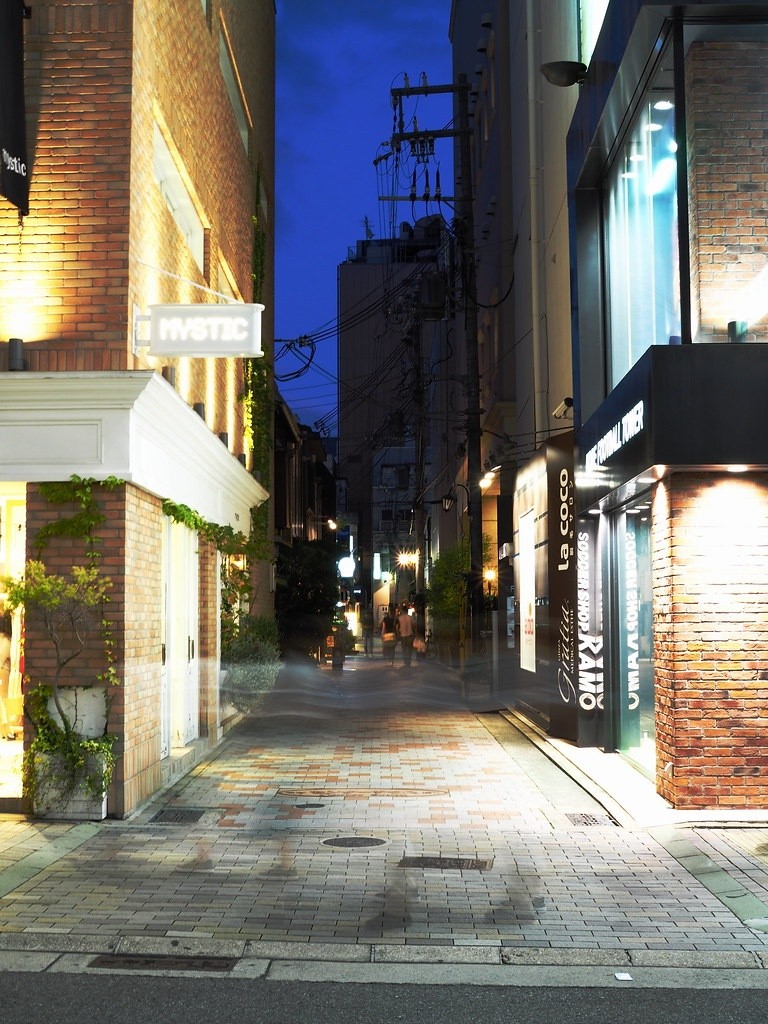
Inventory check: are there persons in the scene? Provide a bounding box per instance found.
[398,605,417,666]
[381,607,401,666]
[360,610,374,658]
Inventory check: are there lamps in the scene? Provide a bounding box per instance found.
[193,402,205,421]
[477,426,573,487]
[219,432,228,449]
[441,484,472,517]
[238,453,246,469]
[8,338,29,371]
[161,366,175,388]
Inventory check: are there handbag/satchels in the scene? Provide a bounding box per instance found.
[412,637,426,653]
[382,632,397,649]
